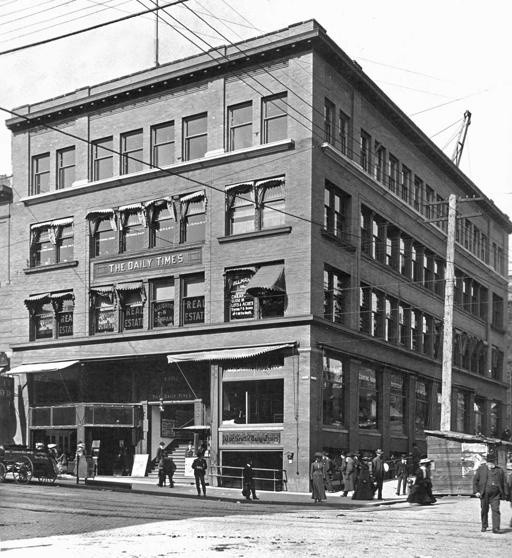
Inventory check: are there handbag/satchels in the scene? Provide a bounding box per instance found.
[242,490,251,497]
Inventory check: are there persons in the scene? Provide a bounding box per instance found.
[158,456,176,488]
[473,453,510,533]
[191,452,206,497]
[310,448,384,503]
[184,444,193,458]
[395,454,409,495]
[407,459,437,505]
[243,459,259,501]
[157,442,166,487]
[197,444,205,457]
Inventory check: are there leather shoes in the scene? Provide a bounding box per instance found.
[492,528,505,534]
[481,526,486,532]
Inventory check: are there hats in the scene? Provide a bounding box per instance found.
[375,449,385,454]
[419,458,434,464]
[487,454,498,462]
[314,452,322,458]
